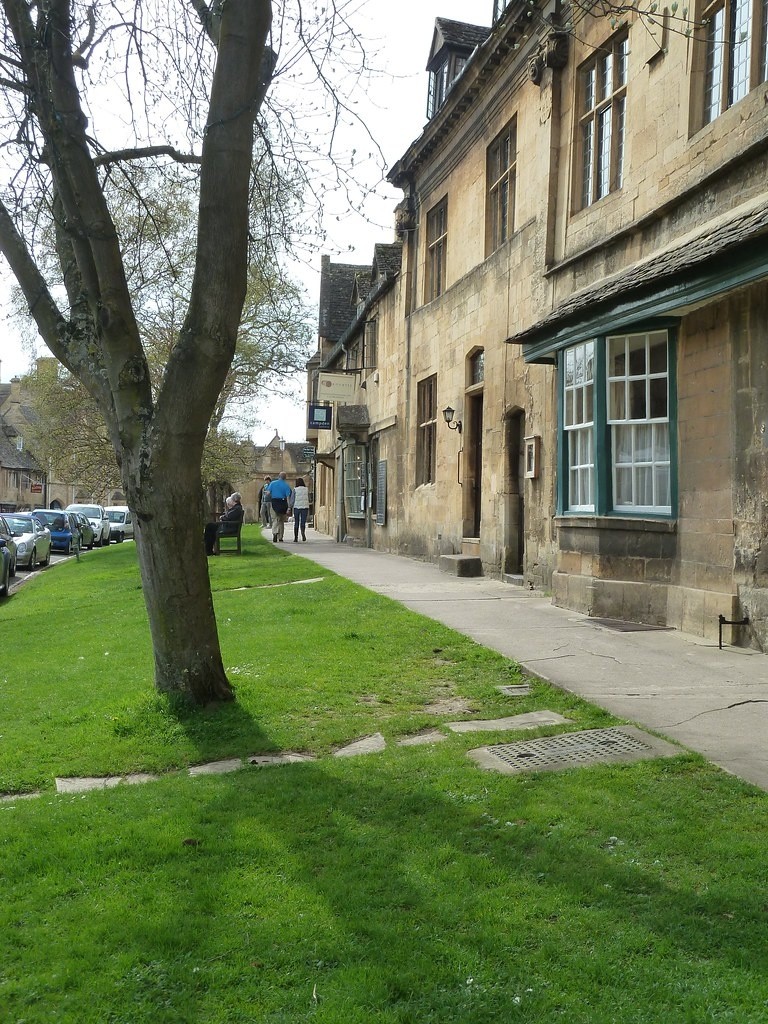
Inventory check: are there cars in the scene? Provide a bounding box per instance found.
[32,509,79,554]
[66,510,97,551]
[0,513,51,571]
[0,537,10,603]
[0,514,17,578]
[64,503,111,548]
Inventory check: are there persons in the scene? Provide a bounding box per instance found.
[260,474,271,529]
[204,492,243,555]
[289,478,309,541]
[267,471,291,541]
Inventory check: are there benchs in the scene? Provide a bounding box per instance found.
[213,510,245,557]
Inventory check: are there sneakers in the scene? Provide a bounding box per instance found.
[279,539,283,542]
[273,533,277,542]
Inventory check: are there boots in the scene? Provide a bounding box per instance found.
[293,527,299,542]
[301,527,307,541]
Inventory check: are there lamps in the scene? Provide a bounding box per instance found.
[441,406,462,433]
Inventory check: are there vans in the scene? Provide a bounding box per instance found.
[102,506,134,544]
